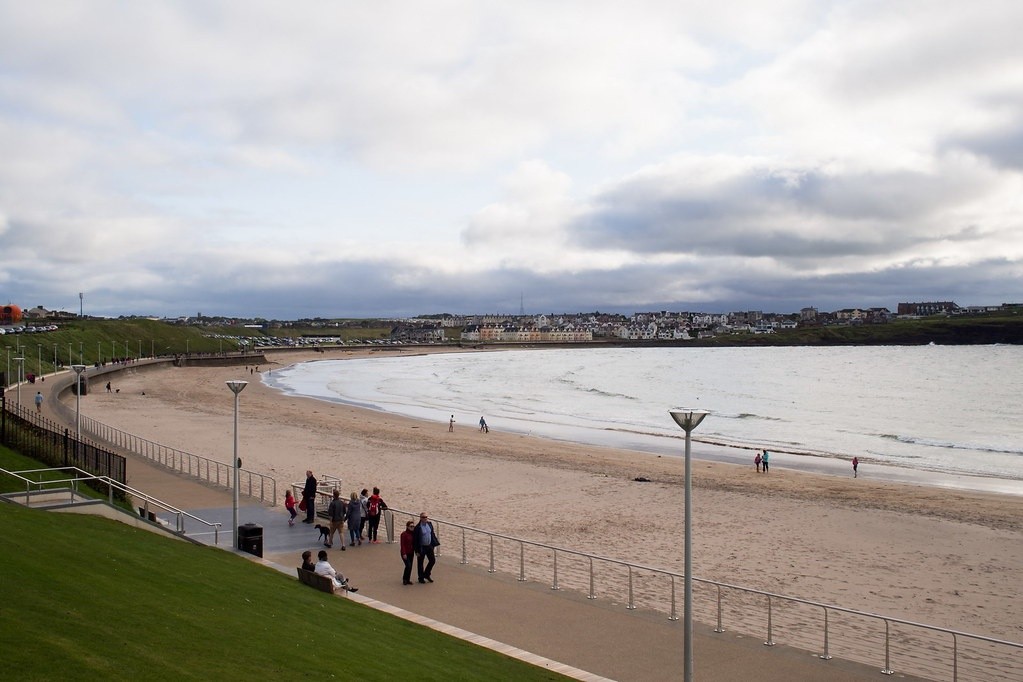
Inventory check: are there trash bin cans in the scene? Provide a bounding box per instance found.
[237,522,263,558]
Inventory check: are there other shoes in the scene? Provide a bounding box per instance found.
[359,537,365,540]
[348,587,359,592]
[369,539,377,544]
[341,546,345,551]
[350,542,355,546]
[288,518,295,526]
[418,579,426,583]
[358,540,362,545]
[324,543,331,548]
[424,575,434,582]
[403,581,414,585]
[303,519,314,524]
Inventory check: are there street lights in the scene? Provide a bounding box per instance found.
[669,408,710,682]
[12,358,25,418]
[72,365,85,468]
[224,380,249,548]
[80,342,83,365]
[6,346,11,387]
[98,342,101,362]
[38,344,42,380]
[126,341,128,358]
[69,343,72,371]
[53,343,57,376]
[79,293,83,318]
[138,340,142,359]
[20,346,26,382]
[112,341,115,359]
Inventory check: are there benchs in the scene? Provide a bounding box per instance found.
[297,567,348,596]
[139,506,171,526]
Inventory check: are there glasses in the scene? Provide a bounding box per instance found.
[421,516,427,519]
[409,526,414,528]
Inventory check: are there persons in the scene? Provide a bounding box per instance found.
[762,449,769,473]
[358,489,369,536]
[479,416,489,433]
[301,550,359,593]
[285,490,299,525]
[246,365,258,374]
[852,456,858,478]
[35,391,43,413]
[95,356,138,370]
[343,492,364,546]
[302,470,317,524]
[106,381,111,392]
[448,415,455,432]
[324,490,347,551]
[414,512,441,583]
[754,453,762,473]
[366,487,387,544]
[400,521,416,584]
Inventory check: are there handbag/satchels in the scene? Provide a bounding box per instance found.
[366,512,370,520]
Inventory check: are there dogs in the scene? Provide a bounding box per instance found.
[315,524,330,541]
[116,389,120,393]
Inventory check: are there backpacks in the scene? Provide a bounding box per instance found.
[369,497,380,515]
[330,500,345,522]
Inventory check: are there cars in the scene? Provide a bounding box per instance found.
[208,334,435,347]
[0,325,58,334]
